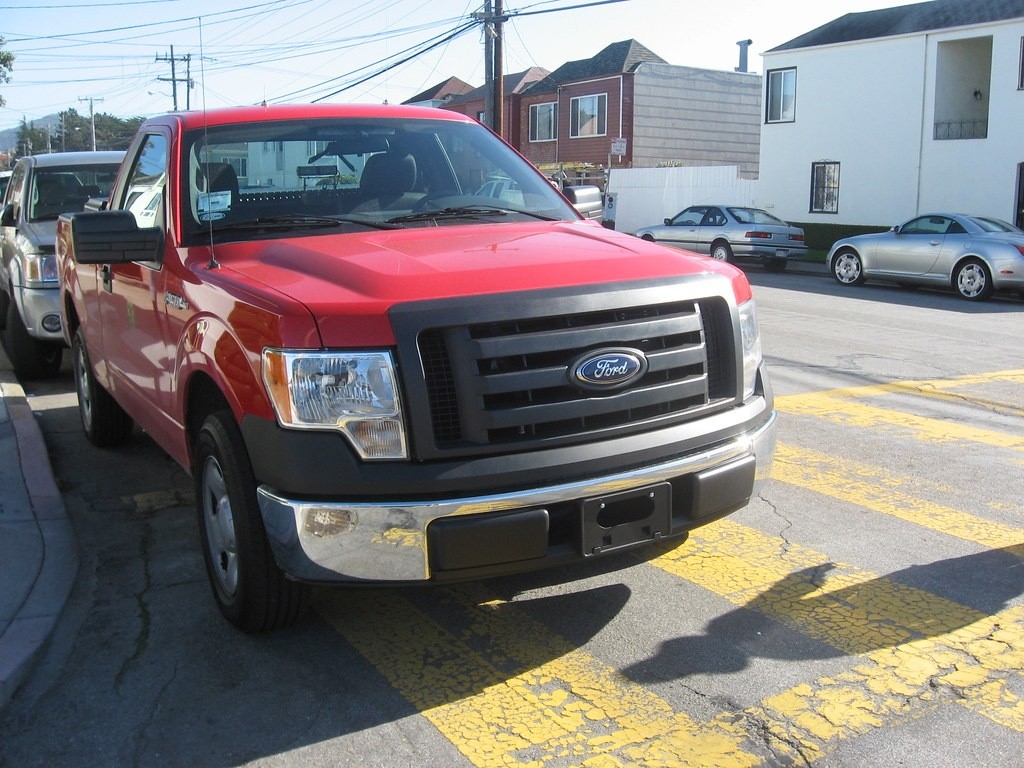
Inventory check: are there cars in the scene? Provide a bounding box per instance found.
[1,169,102,219]
[0,145,147,380]
[826,211,1024,300]
[631,203,810,274]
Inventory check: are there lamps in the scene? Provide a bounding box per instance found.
[973,89,983,100]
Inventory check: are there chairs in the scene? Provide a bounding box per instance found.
[196,162,261,225]
[350,151,427,211]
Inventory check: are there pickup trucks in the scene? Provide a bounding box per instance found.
[54,101,778,637]
